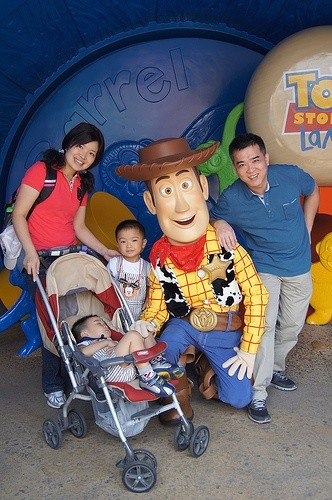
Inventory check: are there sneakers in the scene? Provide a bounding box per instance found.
[44,390,68,409]
[270,371,297,391]
[247,398,272,423]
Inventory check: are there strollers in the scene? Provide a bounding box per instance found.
[28,245,210,492]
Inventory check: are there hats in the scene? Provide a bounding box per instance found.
[115,137,220,182]
[0,224,22,270]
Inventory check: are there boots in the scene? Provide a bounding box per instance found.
[158,345,196,425]
[198,353,255,401]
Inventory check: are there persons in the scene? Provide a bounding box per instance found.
[70,314,185,397]
[10,120,120,409]
[106,219,152,330]
[113,136,270,425]
[207,131,319,425]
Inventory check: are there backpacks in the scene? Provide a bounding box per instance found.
[6,159,57,220]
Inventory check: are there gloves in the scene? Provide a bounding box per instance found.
[222,347,256,380]
[129,319,155,338]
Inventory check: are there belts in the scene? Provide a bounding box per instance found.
[182,308,242,332]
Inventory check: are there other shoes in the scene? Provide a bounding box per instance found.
[151,360,184,379]
[139,371,175,398]
